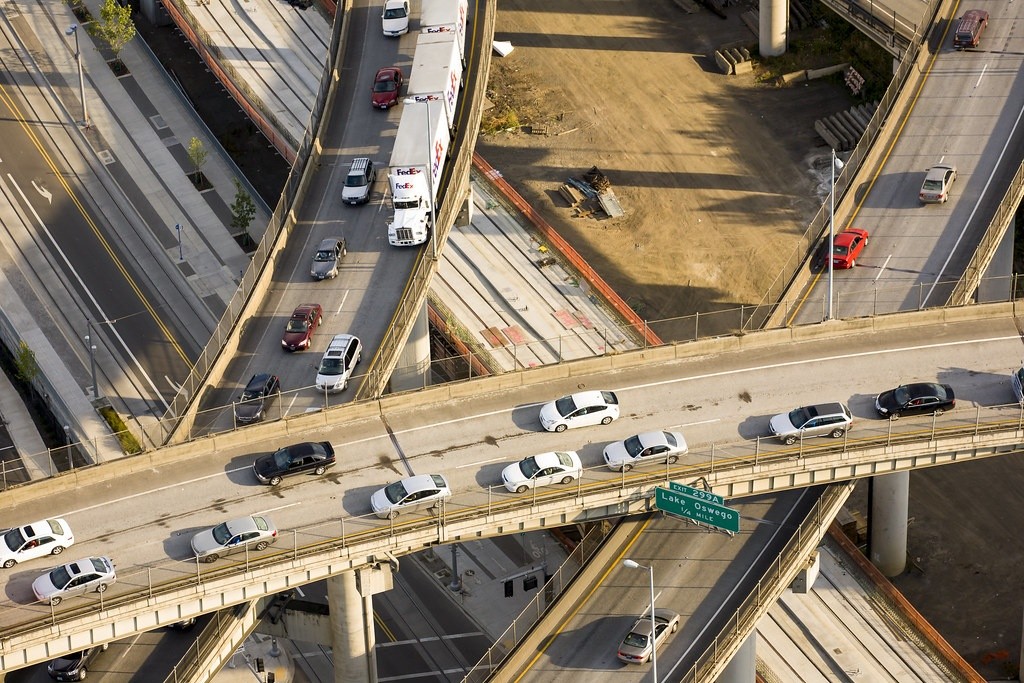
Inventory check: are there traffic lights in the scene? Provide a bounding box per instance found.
[257,657,264,672]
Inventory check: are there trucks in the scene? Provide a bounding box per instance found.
[387,0,468,246]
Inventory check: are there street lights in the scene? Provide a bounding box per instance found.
[828,148,844,322]
[65,23,88,127]
[403,99,437,259]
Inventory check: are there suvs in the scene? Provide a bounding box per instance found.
[768,401,854,445]
[234,373,280,424]
[315,334,362,395]
[342,157,376,205]
[953,9,988,49]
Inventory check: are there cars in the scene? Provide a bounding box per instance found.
[624,559,657,683]
[918,164,957,204]
[48,643,109,683]
[371,474,452,520]
[502,450,583,494]
[539,390,620,433]
[603,431,689,472]
[381,0,410,36]
[0,518,75,568]
[191,515,279,563]
[825,228,868,270]
[370,66,403,110]
[32,556,117,606]
[310,234,347,281]
[281,303,323,352]
[876,383,956,419]
[617,607,680,663]
[253,440,336,486]
